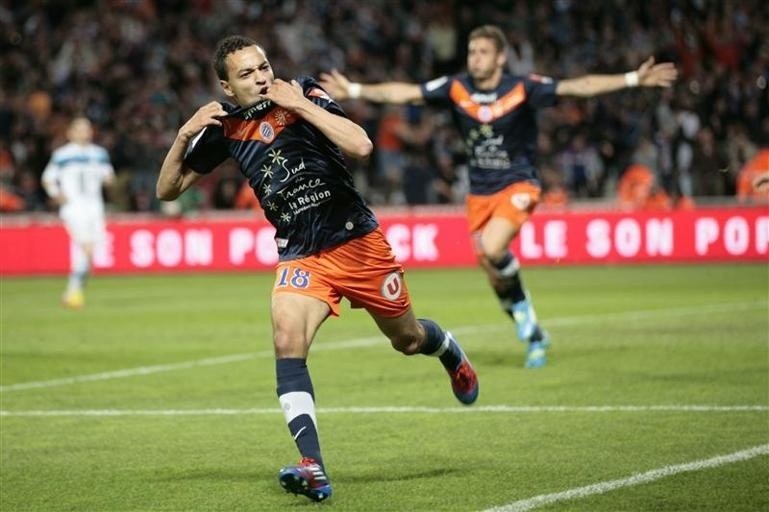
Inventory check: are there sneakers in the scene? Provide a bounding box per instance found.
[510,294,535,340]
[279,458,332,501]
[525,333,548,366]
[447,330,478,404]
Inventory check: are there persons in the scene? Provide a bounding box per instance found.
[155,35,479,504]
[40,117,117,310]
[1,1,768,211]
[316,23,679,369]
